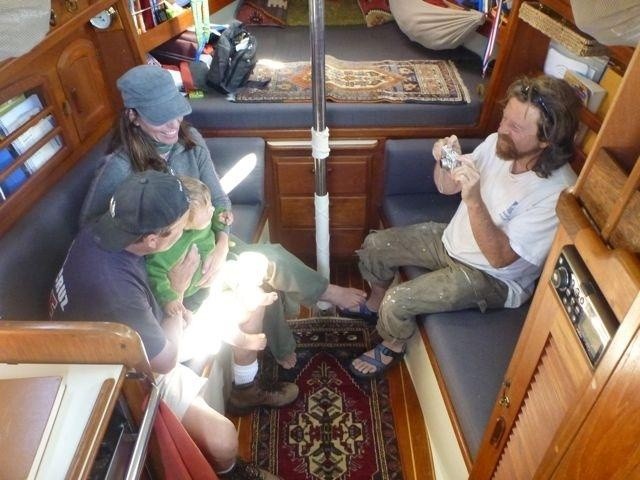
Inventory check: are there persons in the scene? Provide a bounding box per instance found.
[72,61,370,372]
[142,176,279,353]
[332,72,584,381]
[45,167,302,478]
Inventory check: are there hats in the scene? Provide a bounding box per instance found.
[116,65,192,125]
[91,169,189,253]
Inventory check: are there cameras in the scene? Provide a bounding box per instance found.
[440,144,461,175]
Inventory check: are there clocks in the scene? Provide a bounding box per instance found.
[89,6,118,30]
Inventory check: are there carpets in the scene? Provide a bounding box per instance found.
[251,316,404,480]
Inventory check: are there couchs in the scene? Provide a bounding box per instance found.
[376,136,530,469]
[0,126,272,416]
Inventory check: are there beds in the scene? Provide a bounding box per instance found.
[181,24,497,137]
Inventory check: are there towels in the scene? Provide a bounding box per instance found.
[141,394,219,480]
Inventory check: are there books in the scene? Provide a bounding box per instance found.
[0,92,67,206]
[1,373,70,480]
[564,70,608,148]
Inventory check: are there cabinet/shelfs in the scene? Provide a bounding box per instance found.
[467,41,640,480]
[263,140,378,258]
[1,6,132,238]
[0,320,161,480]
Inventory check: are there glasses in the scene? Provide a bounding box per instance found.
[522,83,551,119]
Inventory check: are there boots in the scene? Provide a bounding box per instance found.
[218,456,279,480]
[228,369,299,416]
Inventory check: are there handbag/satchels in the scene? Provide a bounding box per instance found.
[208,20,258,94]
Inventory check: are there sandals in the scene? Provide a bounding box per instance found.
[336,296,378,321]
[349,342,405,379]
